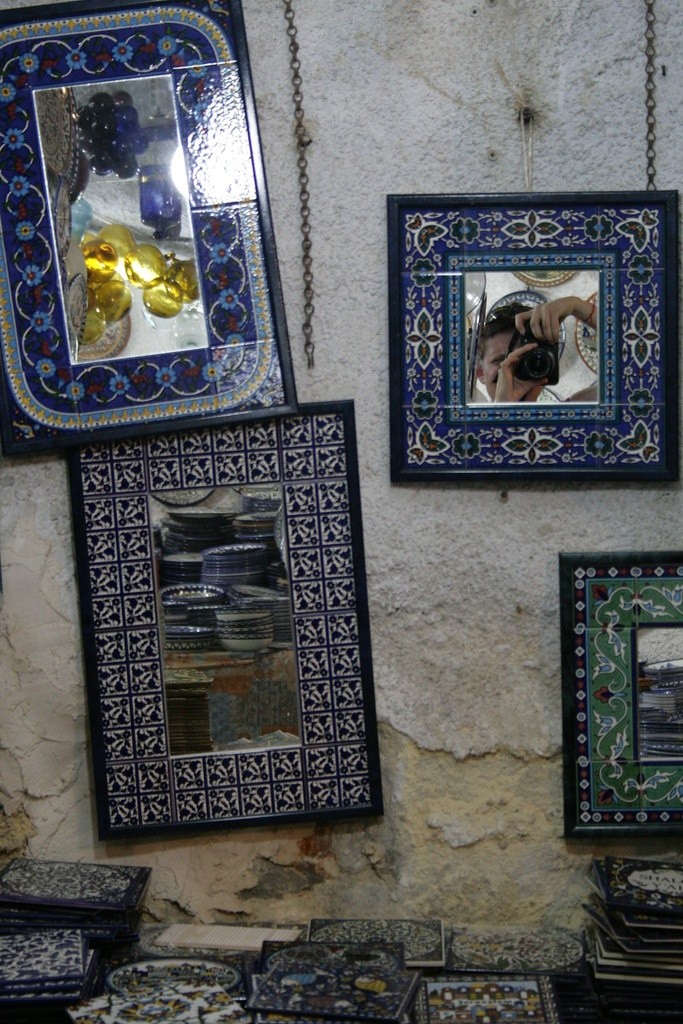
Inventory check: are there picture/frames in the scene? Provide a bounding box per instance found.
[0,856,683,1024]
[383,192,681,485]
[0,0,301,451]
[559,549,682,841]
[67,399,385,840]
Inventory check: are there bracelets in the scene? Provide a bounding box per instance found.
[583,303,595,322]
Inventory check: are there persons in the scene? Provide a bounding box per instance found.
[475,296,599,402]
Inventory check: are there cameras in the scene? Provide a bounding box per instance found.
[505,334,560,386]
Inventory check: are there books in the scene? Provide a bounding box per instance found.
[580,854,683,985]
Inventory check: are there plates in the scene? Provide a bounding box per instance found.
[509,271,576,287]
[638,658,683,760]
[574,292,600,376]
[36,88,130,363]
[148,483,302,755]
[0,857,683,1024]
[464,271,486,315]
[485,290,566,362]
[464,317,473,379]
[536,388,560,402]
